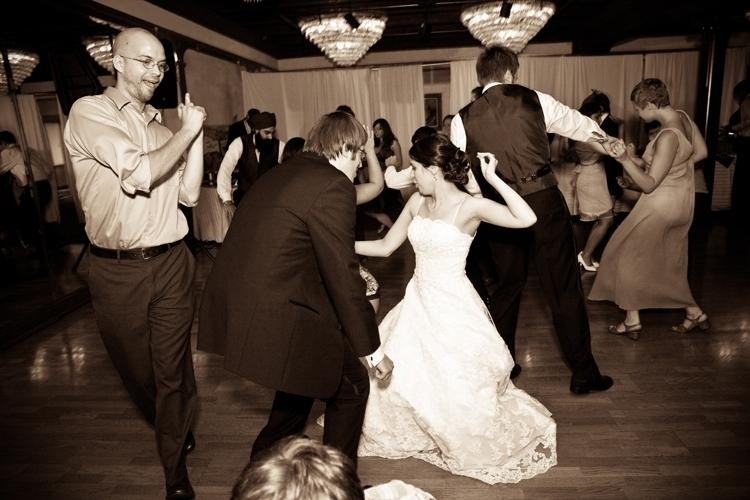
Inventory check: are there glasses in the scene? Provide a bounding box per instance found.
[359,146,368,159]
[121,54,170,72]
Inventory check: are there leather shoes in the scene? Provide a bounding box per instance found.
[165,478,196,499]
[569,373,614,394]
[510,363,521,378]
[183,431,195,454]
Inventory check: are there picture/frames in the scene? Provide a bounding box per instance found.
[424,93,443,132]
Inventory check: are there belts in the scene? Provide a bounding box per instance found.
[84,241,181,261]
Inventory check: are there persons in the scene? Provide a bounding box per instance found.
[569,93,662,272]
[0,131,53,271]
[587,79,711,341]
[316,134,558,483]
[61,28,207,500]
[197,111,394,472]
[338,105,455,233]
[719,80,749,226]
[215,109,304,220]
[230,434,435,500]
[448,46,621,396]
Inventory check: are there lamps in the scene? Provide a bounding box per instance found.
[0,49,39,96]
[82,36,118,77]
[457,0,554,57]
[297,1,388,67]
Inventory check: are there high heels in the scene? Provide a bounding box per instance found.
[578,252,597,272]
[672,311,710,333]
[579,251,600,268]
[607,320,642,340]
